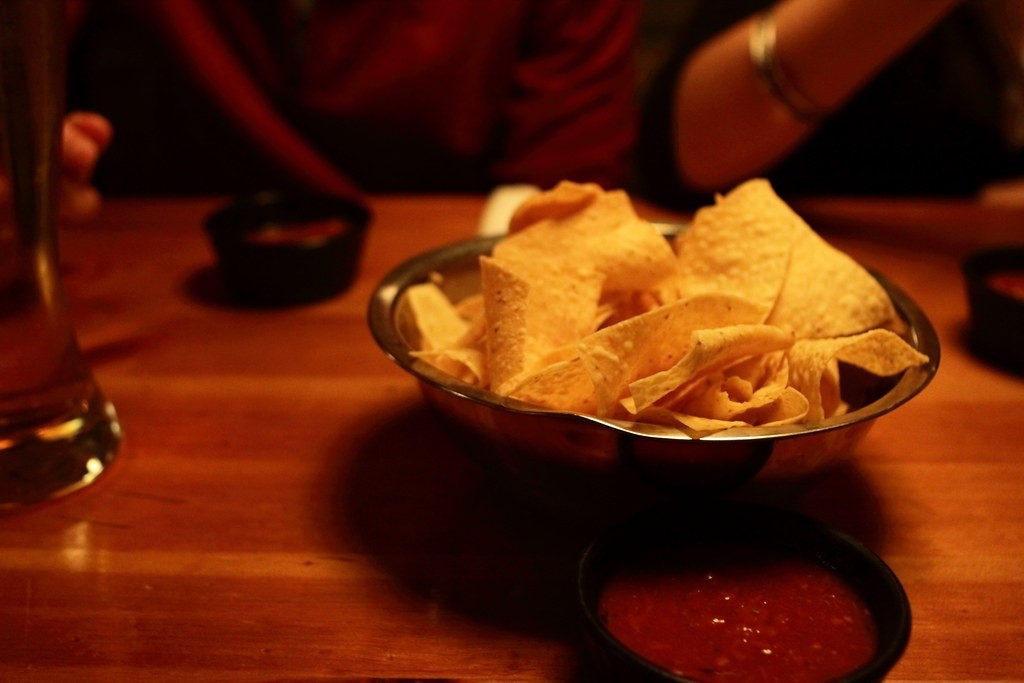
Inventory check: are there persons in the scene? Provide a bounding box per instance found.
[290,0,648,203]
[636,0,1024,210]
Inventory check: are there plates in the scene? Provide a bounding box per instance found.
[368,222,948,492]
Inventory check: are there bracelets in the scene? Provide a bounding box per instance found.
[751,9,824,124]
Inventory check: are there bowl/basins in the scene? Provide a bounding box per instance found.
[578,508,914,683]
[957,242,1024,366]
[197,187,370,310]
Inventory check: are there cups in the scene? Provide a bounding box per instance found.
[0,0,120,515]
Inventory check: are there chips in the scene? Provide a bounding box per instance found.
[399,177,932,431]
